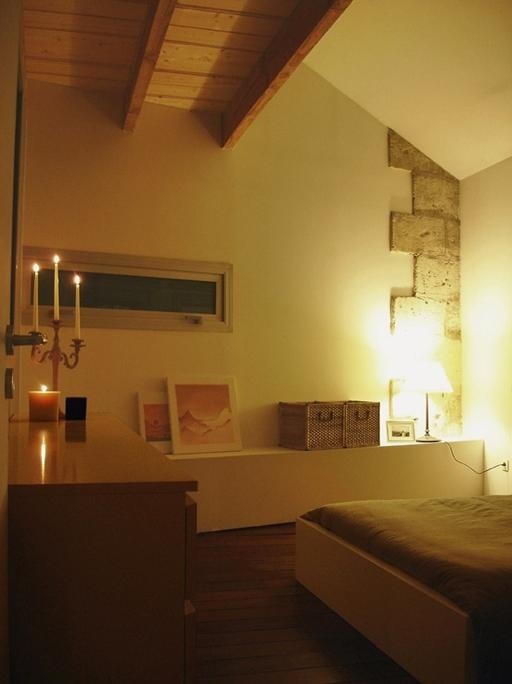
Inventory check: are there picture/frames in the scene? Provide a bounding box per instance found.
[386,421,415,442]
[138,375,243,455]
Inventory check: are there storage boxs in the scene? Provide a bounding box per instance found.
[279,401,380,450]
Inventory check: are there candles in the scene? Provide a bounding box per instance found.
[28,390,60,421]
[32,254,85,341]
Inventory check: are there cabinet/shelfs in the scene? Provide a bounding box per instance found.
[0,416,197,684]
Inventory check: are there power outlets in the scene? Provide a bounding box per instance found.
[502,459,509,472]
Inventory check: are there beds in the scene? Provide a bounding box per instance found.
[296,494,511,684]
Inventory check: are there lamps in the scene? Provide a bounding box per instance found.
[407,351,453,442]
